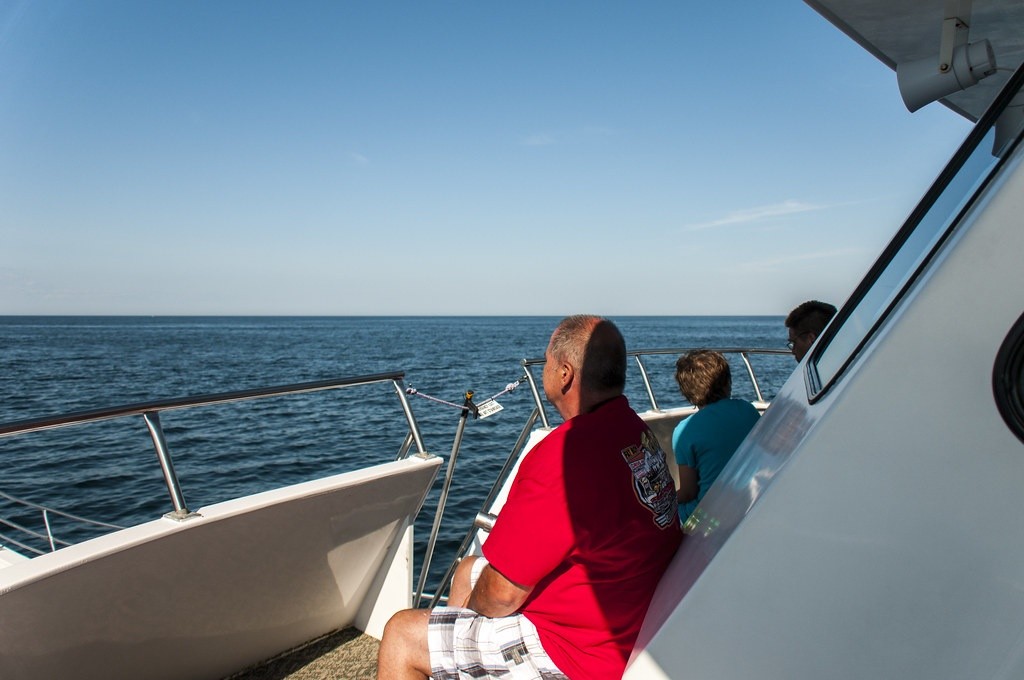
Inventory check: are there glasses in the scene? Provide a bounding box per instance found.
[787,337,800,350]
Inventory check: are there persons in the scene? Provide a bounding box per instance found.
[784,300,837,362]
[673,347,759,524]
[376,313,684,680]
